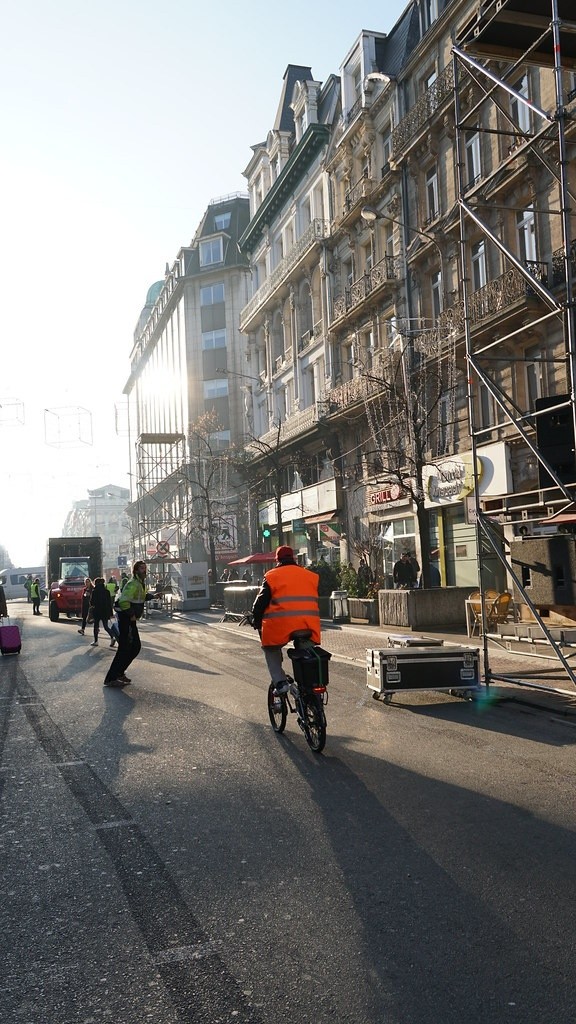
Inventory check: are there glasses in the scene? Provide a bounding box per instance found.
[404,556,407,557]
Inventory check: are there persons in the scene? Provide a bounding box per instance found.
[407,552,420,573]
[104,561,164,687]
[90,577,116,647]
[393,553,415,588]
[0,585,8,620]
[243,570,252,584]
[358,559,373,583]
[24,576,33,602]
[78,578,95,635]
[120,572,128,593]
[31,578,43,615]
[247,544,321,696]
[105,577,118,603]
[155,579,163,593]
[419,560,441,588]
[348,562,356,574]
[207,569,238,584]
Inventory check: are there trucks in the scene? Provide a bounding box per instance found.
[44,535,103,622]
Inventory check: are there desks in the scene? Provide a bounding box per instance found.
[465,598,506,638]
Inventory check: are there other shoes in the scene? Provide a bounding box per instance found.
[90,641,98,646]
[118,675,131,682]
[78,630,84,635]
[33,612,37,615]
[104,681,125,687]
[272,683,288,695]
[110,637,116,647]
[37,612,42,615]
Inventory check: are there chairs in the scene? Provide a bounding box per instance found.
[465,590,513,637]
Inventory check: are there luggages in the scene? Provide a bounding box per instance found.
[109,617,120,643]
[0,616,21,655]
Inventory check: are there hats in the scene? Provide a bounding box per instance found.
[134,561,145,571]
[274,546,294,559]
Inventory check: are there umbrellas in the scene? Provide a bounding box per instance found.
[228,553,298,573]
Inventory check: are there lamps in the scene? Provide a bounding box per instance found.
[361,206,456,311]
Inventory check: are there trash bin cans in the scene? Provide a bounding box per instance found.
[329,590,351,624]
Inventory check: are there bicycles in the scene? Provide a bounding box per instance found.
[237,610,331,755]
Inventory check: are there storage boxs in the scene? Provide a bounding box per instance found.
[365,647,480,705]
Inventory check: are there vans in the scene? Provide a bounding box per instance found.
[0,565,46,601]
